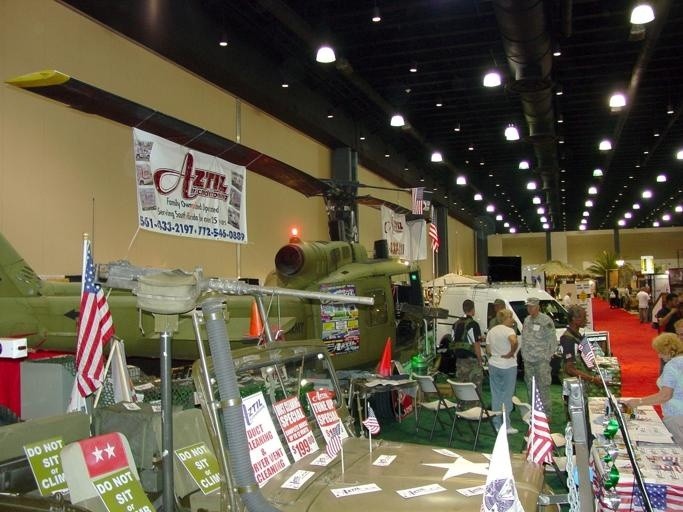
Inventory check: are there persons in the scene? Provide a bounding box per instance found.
[656,293,682,377]
[619,332,682,447]
[562,291,572,305]
[558,302,605,424]
[484,308,519,434]
[521,296,558,424]
[606,282,683,339]
[487,299,518,337]
[450,299,486,422]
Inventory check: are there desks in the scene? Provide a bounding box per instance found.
[587,395,682,511]
[335,373,417,428]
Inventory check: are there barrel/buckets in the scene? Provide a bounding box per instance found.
[411,352,428,376]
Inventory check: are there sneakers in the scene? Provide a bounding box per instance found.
[507,426,518,434]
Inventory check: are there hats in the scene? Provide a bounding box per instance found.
[495,298,505,305]
[524,298,539,305]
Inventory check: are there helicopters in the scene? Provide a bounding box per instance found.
[0,66,425,376]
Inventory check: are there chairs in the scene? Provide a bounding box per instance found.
[394,359,419,429]
[410,370,502,452]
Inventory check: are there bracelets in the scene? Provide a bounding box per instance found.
[637,397,643,406]
[588,374,595,384]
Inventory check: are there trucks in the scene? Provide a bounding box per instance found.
[427,285,584,375]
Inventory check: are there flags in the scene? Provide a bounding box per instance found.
[577,334,595,370]
[411,186,424,218]
[524,376,555,465]
[427,205,439,255]
[600,477,682,512]
[74,240,115,399]
[361,406,380,435]
[322,419,342,457]
[480,421,523,512]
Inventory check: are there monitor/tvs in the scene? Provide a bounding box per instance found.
[485,255,522,283]
